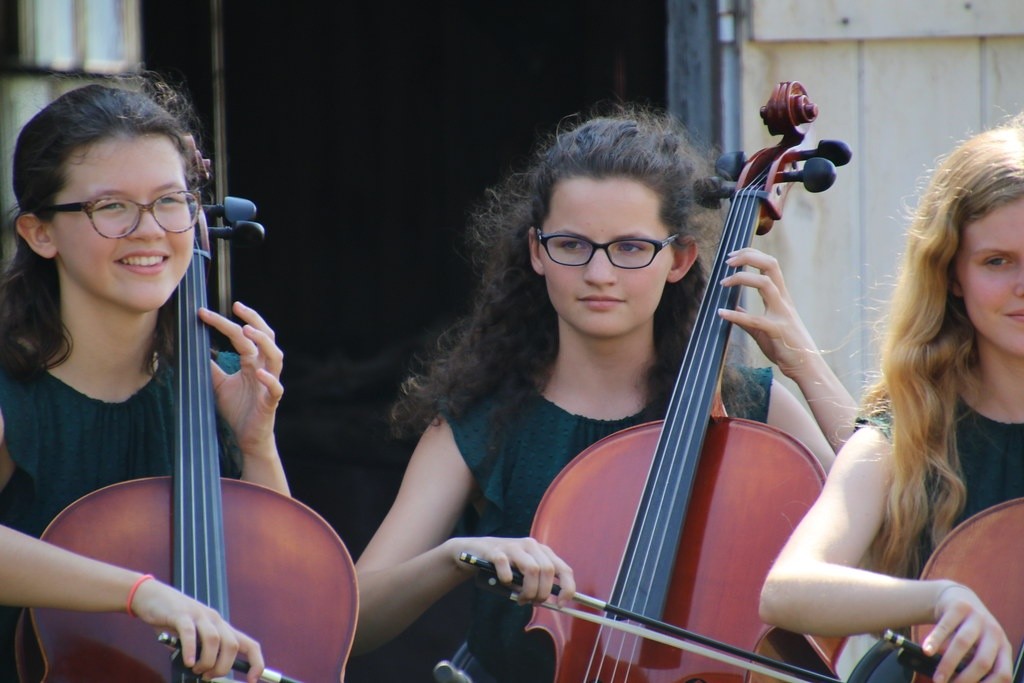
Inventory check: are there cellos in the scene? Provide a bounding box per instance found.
[14,61,360,683]
[909,497,1024,683]
[524,78,849,683]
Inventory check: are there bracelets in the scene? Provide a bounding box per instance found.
[932,582,977,619]
[127,568,154,618]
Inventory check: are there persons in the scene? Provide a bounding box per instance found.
[759,115,1024,682]
[0,75,298,683]
[353,101,859,681]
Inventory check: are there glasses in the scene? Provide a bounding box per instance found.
[536,228,680,269]
[53,190,200,239]
